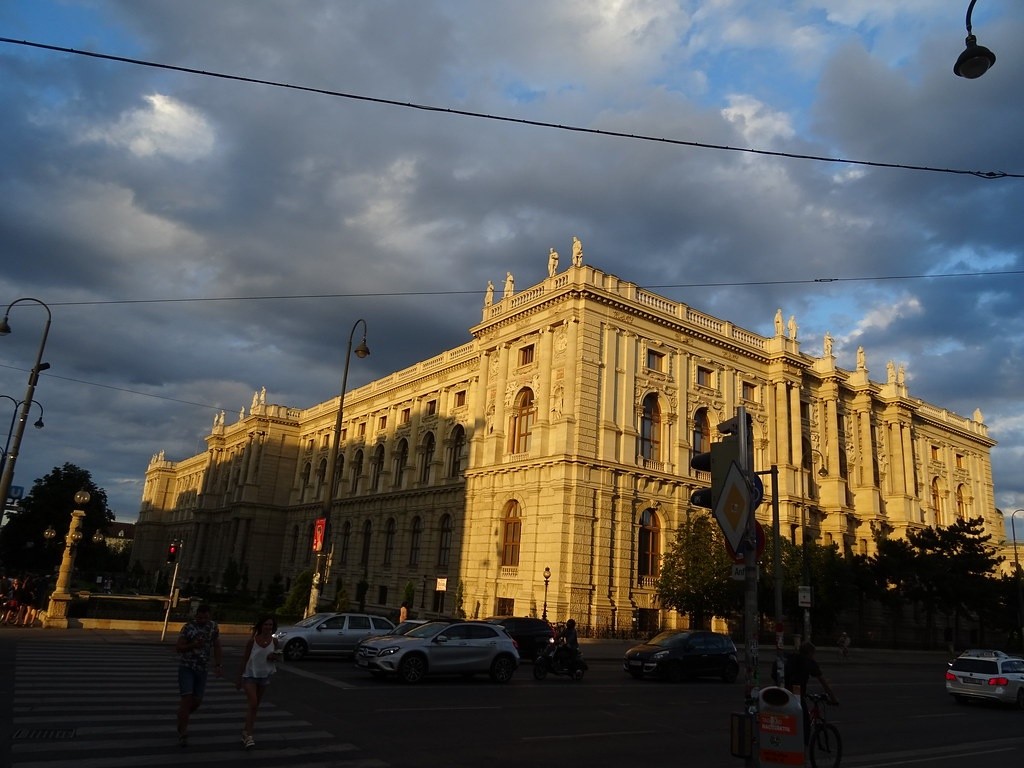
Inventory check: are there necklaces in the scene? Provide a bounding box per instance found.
[263,639,270,644]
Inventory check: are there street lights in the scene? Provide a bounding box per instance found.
[0,298,53,524]
[42,492,104,630]
[542,567,551,621]
[801,448,828,643]
[0,394,45,481]
[307,319,370,614]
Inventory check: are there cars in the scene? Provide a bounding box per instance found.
[481,616,556,663]
[945,648,1024,710]
[271,611,397,662]
[353,621,522,685]
[623,629,740,684]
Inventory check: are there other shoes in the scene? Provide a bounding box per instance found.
[0,621,35,628]
[178,735,187,748]
[570,671,577,679]
[176,712,181,732]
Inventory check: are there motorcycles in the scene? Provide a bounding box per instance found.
[533,635,587,680]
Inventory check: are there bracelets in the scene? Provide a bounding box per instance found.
[215,664,224,667]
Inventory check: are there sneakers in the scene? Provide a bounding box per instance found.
[240,730,255,751]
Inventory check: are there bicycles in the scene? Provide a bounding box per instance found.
[806,691,842,768]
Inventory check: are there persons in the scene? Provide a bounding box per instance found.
[838,631,850,663]
[251,391,258,408]
[548,248,559,278]
[774,309,799,341]
[214,409,225,425]
[823,331,834,356]
[400,601,408,624]
[260,387,266,404]
[770,641,830,755]
[501,272,514,299]
[239,406,245,420]
[886,359,905,385]
[103,576,113,593]
[0,573,48,628]
[484,281,494,307]
[572,237,583,267]
[151,449,164,464]
[856,346,868,370]
[745,632,761,684]
[176,604,224,744]
[235,615,284,748]
[552,620,580,678]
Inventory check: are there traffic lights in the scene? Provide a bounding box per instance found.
[689,442,738,517]
[168,545,176,561]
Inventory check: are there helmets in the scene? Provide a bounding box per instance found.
[566,619,575,627]
